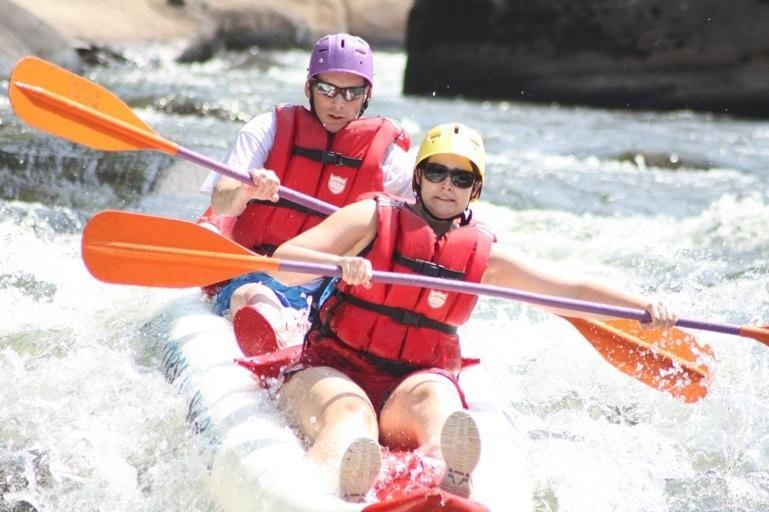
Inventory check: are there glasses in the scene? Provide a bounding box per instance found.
[420,160,476,188]
[310,76,370,101]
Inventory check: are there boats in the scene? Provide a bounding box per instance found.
[103,288,489,512]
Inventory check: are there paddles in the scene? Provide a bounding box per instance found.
[81,210,769,347]
[8,56,717,403]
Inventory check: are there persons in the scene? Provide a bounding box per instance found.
[269,120,680,505]
[198,32,417,360]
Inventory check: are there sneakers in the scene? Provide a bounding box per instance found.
[440,409,481,498]
[232,307,287,357]
[339,440,383,503]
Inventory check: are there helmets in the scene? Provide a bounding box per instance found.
[308,32,373,97]
[412,122,486,202]
